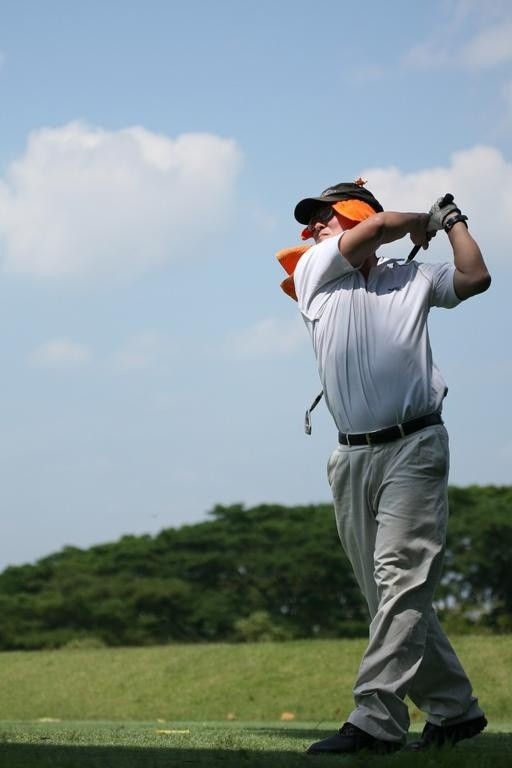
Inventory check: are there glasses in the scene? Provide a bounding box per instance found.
[306,207,335,231]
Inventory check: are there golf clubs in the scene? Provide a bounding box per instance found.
[304,194,454,435]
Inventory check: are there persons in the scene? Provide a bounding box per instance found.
[273,177,493,757]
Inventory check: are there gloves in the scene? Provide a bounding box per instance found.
[421,192,458,233]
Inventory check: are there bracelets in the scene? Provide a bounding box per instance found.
[444,216,468,232]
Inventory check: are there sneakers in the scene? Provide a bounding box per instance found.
[307,719,405,756]
[406,703,489,752]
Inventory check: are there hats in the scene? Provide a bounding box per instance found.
[293,178,383,224]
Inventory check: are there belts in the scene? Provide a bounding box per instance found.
[336,414,445,447]
